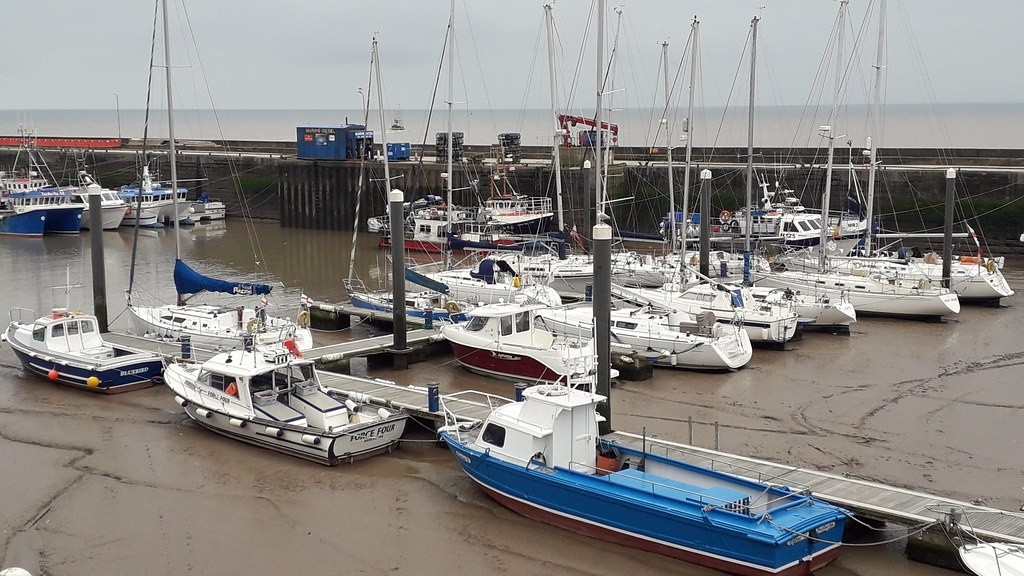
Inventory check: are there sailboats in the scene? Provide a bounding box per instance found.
[126,0,313,351]
[343,1,1015,387]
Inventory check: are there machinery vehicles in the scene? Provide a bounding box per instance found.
[558,114,618,148]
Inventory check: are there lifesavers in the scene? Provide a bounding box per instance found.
[721,211,731,222]
[299,311,310,324]
[248,319,261,334]
[987,260,994,271]
[446,301,459,313]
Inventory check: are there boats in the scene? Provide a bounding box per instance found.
[162,348,410,466]
[437,317,855,576]
[60,148,129,228]
[0,115,87,237]
[187,192,226,221]
[958,543,1024,576]
[118,161,195,229]
[0,307,162,395]
